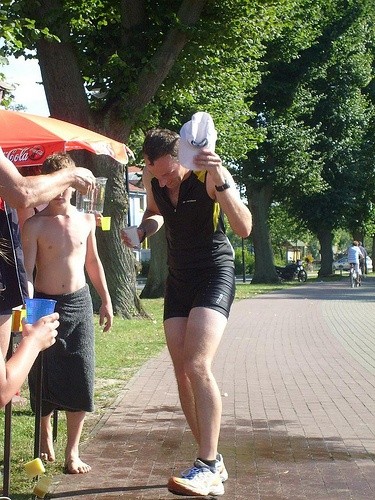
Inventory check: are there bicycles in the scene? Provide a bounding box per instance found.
[347,258,364,288]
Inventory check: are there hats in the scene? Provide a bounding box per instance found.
[177,112,217,170]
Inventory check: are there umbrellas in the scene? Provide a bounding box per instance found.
[0,108,136,167]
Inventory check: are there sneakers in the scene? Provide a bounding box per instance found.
[214,454,228,482]
[167,460,224,496]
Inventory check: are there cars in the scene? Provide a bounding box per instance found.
[332,254,373,274]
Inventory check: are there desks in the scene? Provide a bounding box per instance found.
[0,313,48,500]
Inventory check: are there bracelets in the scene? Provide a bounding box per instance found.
[137,227,146,244]
[215,180,231,192]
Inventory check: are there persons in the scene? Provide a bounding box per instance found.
[0,144,97,415]
[347,241,364,285]
[355,241,368,276]
[20,152,116,474]
[120,128,252,496]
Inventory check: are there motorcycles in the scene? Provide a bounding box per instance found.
[276,259,308,283]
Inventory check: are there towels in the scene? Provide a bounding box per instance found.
[31,283,96,417]
